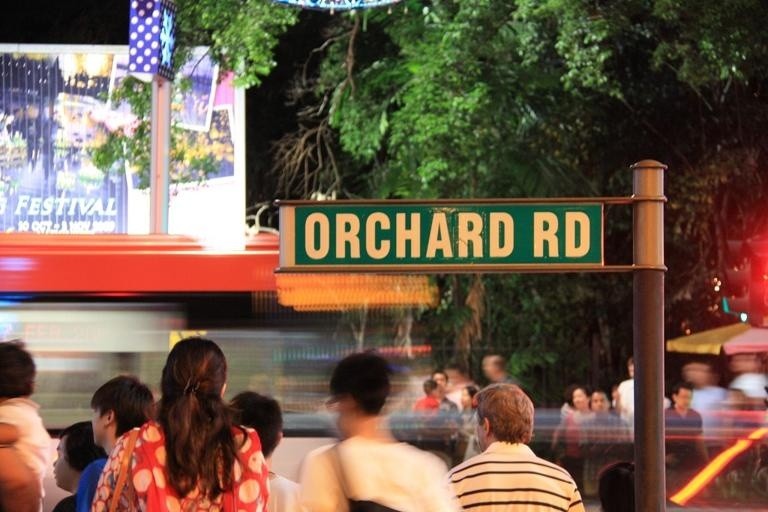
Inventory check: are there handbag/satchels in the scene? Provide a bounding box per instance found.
[110,490,138,512]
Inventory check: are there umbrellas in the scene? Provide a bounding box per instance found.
[666,322,768,356]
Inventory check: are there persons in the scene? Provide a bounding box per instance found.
[0,337,768,512]
